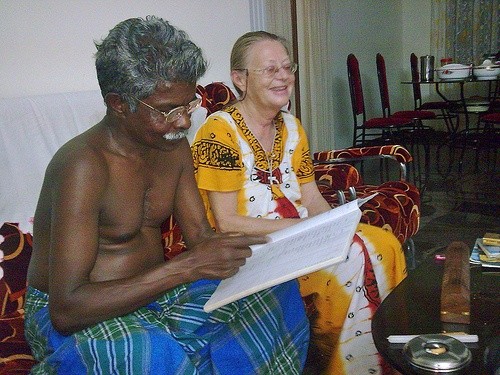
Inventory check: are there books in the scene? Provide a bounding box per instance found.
[469,233,500,268]
[204,192,379,314]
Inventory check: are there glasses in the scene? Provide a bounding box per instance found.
[122,86,203,123]
[234,63,298,77]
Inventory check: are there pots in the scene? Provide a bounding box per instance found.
[473,65,500,80]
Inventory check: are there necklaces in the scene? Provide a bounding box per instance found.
[238,106,281,185]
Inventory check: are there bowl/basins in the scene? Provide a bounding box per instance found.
[435,64,472,81]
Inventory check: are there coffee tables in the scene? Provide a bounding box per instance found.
[371,241,500,375]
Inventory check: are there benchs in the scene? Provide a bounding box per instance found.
[0,81,421,375]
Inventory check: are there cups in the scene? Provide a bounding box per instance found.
[440,58,452,66]
[421,55,434,81]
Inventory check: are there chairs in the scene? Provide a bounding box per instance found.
[346,53,500,184]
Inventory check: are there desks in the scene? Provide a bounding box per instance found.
[400,78,500,170]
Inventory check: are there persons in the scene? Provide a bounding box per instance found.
[25,16,311,375]
[188,31,410,375]
